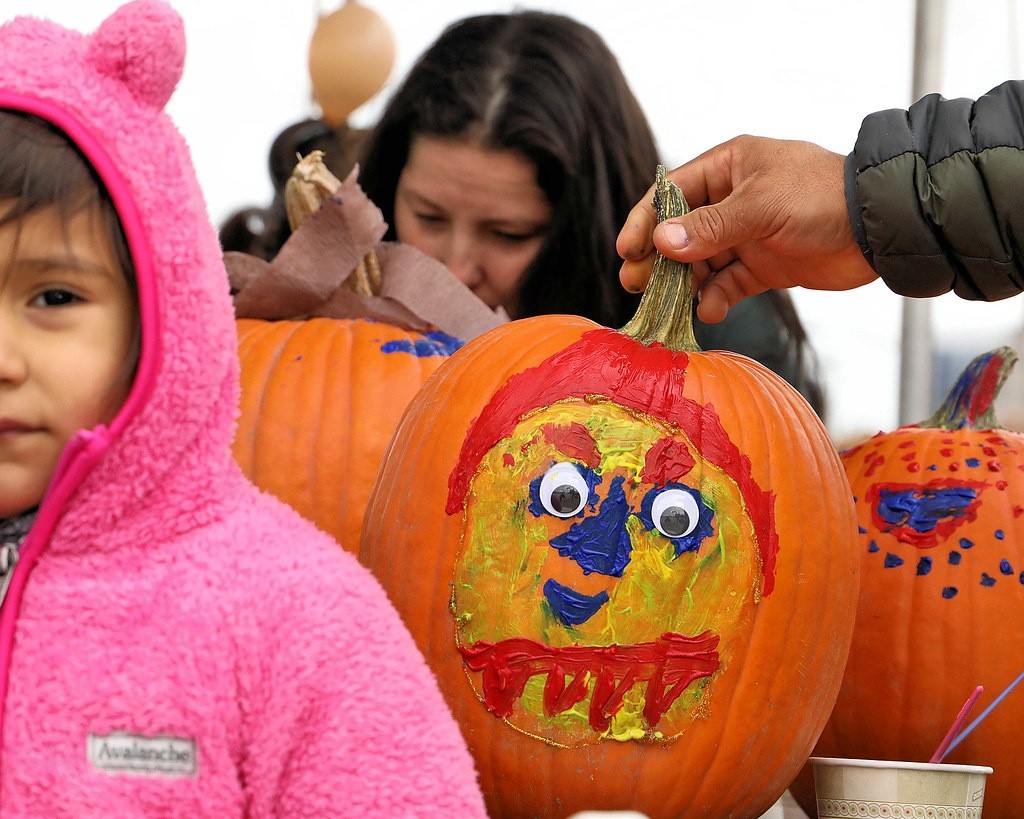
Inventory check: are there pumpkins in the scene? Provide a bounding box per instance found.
[784,345,1024,819]
[226,155,461,557]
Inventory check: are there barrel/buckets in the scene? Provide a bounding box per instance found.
[809,756,994,819]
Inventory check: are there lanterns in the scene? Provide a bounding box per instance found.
[791,347,1023,819]
[358,162,863,819]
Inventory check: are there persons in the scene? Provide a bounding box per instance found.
[0,0,491,819]
[615,73,1024,325]
[350,7,828,429]
